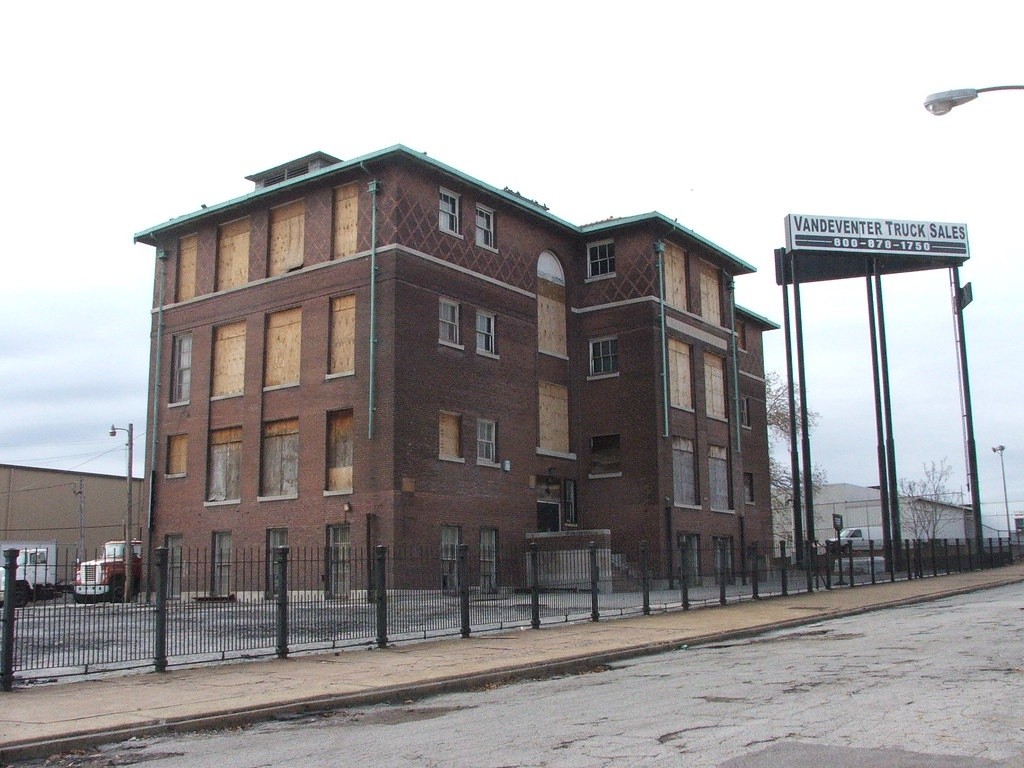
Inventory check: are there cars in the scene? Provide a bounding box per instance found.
[71,540,142,604]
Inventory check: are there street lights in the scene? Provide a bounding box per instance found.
[992,446,1013,565]
[110,423,133,603]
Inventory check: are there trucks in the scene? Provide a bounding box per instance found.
[0,541,63,608]
[825,527,884,554]
